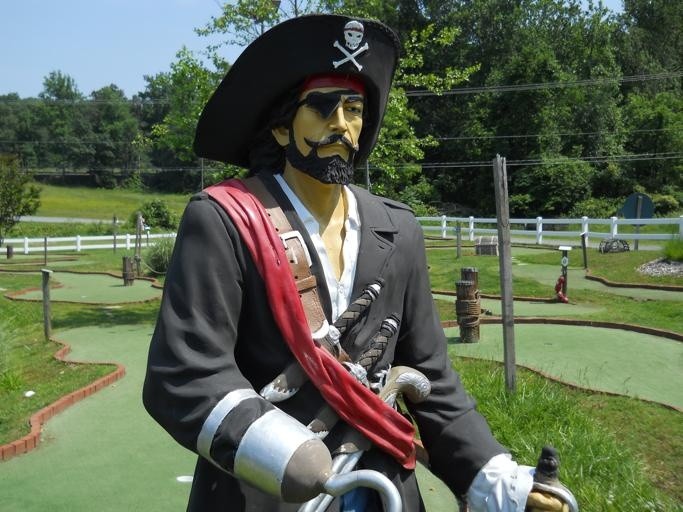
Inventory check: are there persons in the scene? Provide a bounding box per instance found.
[139,13,579,510]
